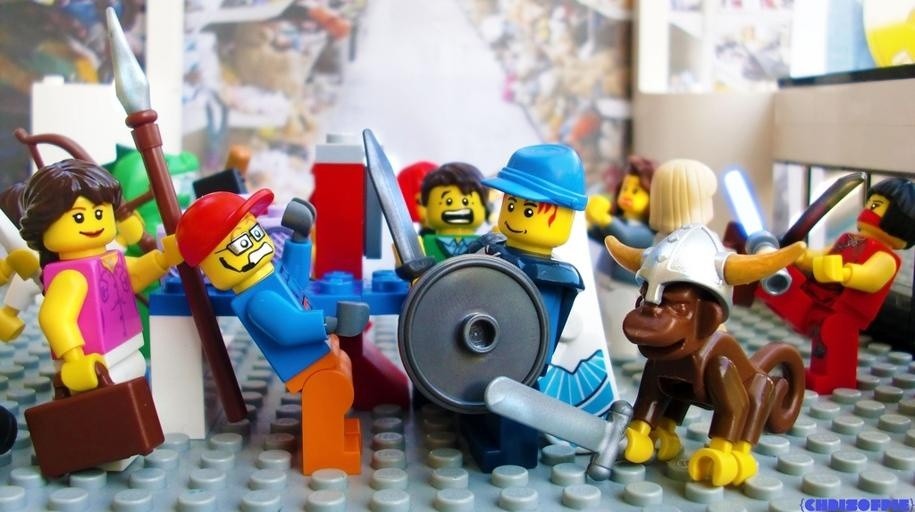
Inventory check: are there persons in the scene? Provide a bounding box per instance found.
[0,143,915,491]
